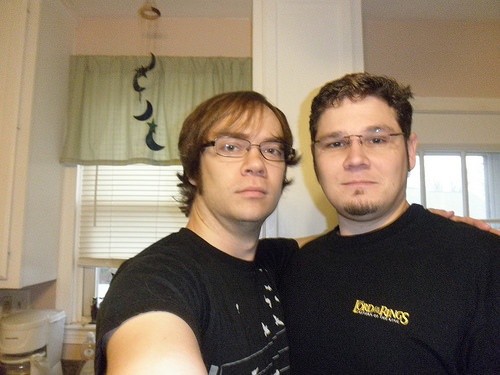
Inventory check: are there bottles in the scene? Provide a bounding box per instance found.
[91,297,99,323]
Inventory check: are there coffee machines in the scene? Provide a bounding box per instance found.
[0,308,66,375]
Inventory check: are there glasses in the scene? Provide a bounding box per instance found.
[314,129,406,152]
[201,136,296,162]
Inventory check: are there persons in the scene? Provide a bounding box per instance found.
[278,72,500,375]
[94,91,500,375]
